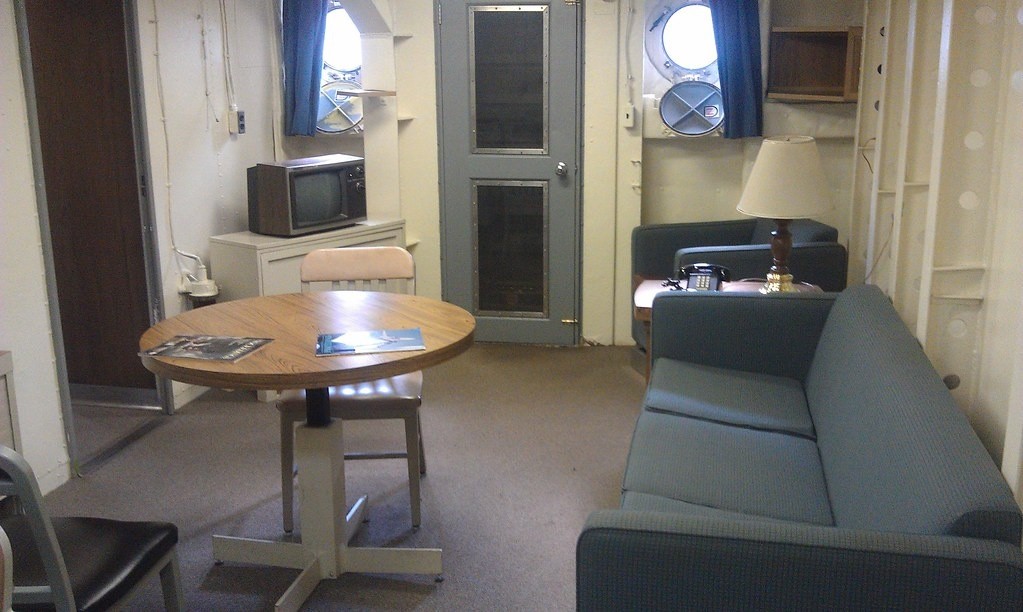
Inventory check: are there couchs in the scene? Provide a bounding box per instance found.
[577,283,1023,612]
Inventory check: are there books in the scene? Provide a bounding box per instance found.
[137,333,276,364]
[314,327,426,357]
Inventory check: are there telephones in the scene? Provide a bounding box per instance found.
[677,262,731,291]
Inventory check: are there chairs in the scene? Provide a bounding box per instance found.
[274,247,427,534]
[0,525,12,612]
[1,445,184,612]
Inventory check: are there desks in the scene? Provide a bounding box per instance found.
[138,291,475,612]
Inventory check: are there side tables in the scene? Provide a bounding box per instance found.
[633,279,827,390]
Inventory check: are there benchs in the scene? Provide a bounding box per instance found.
[630,215,848,351]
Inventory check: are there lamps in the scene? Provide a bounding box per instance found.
[644,0,720,86]
[735,137,830,293]
[322,6,362,73]
[208,220,407,402]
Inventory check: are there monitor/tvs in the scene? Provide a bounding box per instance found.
[246,152,367,237]
